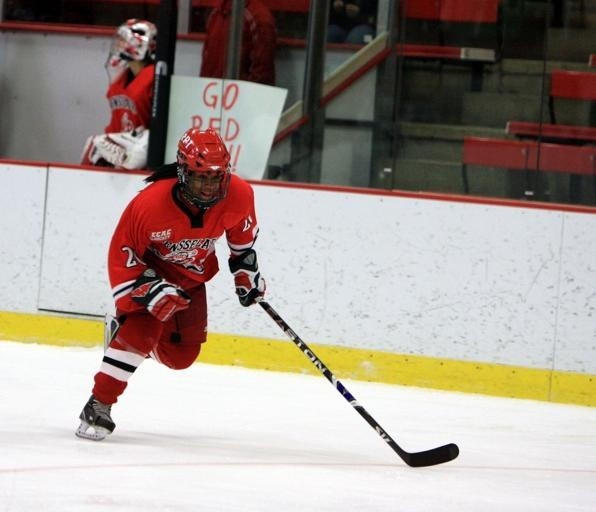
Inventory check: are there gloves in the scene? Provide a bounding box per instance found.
[129,268,193,323]
[228,250,267,307]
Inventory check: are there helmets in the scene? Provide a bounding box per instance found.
[175,125,232,210]
[111,18,158,61]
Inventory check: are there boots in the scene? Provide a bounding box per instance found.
[79,393,116,433]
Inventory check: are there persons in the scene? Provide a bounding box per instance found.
[199,1,280,87]
[324,0,376,46]
[81,18,159,172]
[75,127,269,441]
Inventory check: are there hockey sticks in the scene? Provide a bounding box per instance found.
[257,296,460,468]
